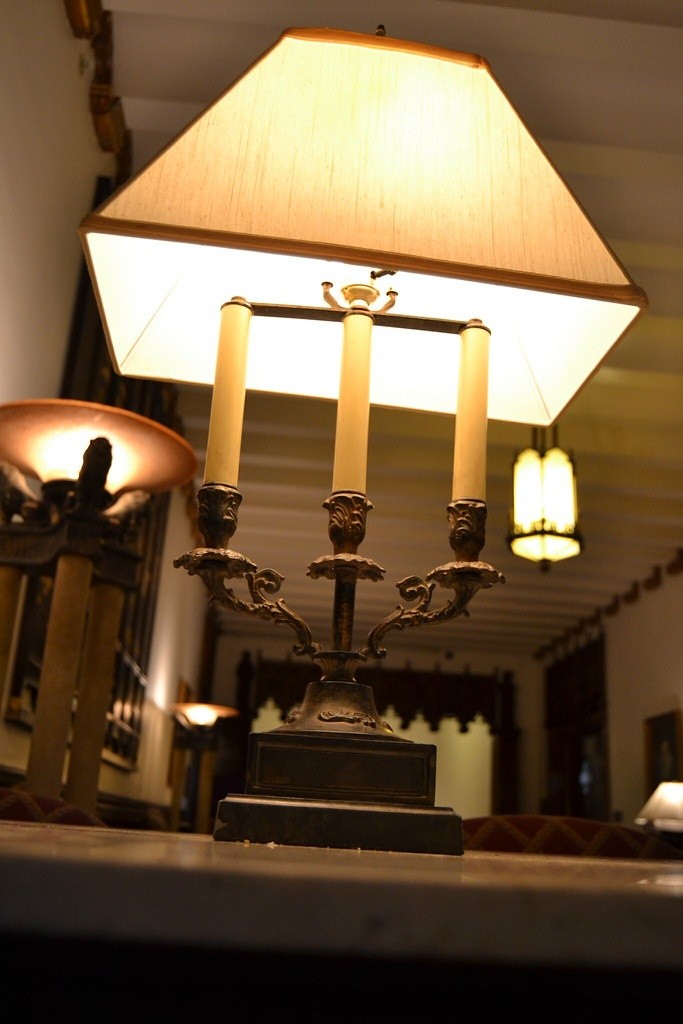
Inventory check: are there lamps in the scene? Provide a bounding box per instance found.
[172,702,240,751]
[78,25,650,856]
[635,781,683,832]
[0,399,198,825]
[507,424,584,573]
